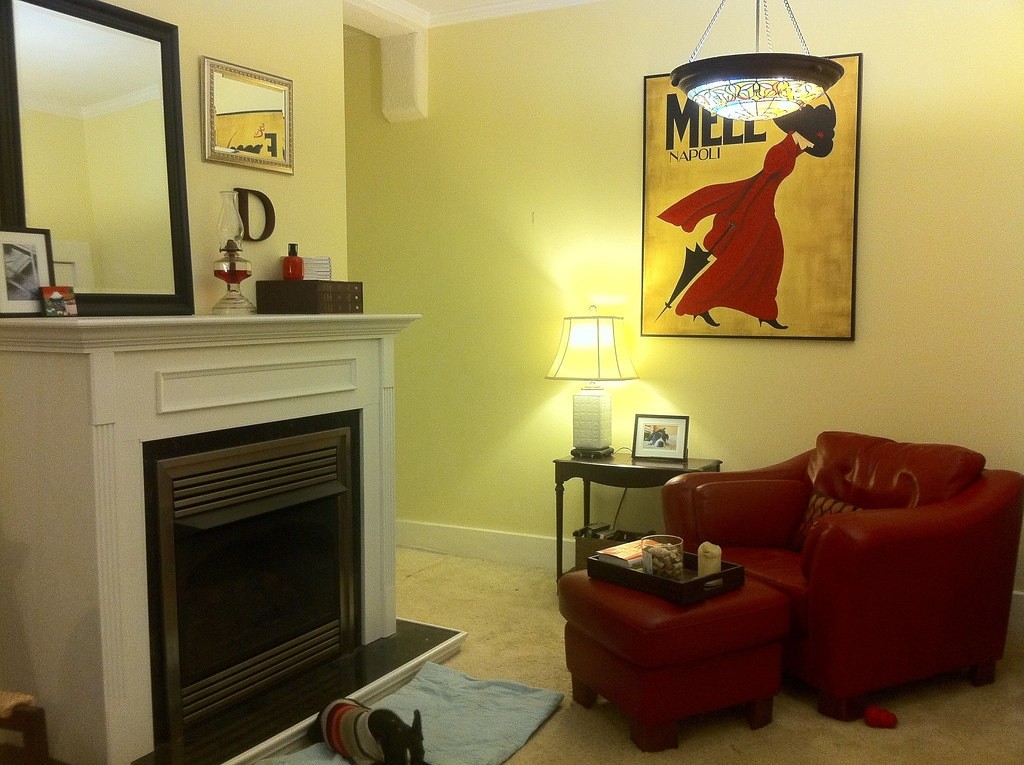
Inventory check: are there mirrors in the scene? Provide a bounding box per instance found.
[0,0,194,314]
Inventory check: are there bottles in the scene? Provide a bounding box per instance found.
[284,243,304,280]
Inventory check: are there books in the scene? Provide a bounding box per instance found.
[596,539,662,568]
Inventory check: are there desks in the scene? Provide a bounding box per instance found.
[553,453,723,593]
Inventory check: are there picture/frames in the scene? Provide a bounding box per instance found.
[0,226,56,318]
[640,53,863,341]
[198,54,296,173]
[632,414,689,463]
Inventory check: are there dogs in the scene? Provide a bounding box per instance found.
[306,698,431,765]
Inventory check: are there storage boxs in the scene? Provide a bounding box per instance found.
[586,537,745,607]
[258,279,364,314]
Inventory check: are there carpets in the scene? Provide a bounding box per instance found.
[252,661,566,765]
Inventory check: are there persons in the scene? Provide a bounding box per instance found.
[648,425,669,447]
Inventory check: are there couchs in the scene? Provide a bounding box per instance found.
[659,431,1024,722]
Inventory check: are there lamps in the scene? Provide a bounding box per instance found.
[544,306,638,458]
[669,0,845,122]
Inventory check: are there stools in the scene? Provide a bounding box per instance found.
[558,568,791,752]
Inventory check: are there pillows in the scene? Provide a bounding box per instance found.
[788,430,967,553]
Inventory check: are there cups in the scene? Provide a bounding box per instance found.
[640,534,683,582]
